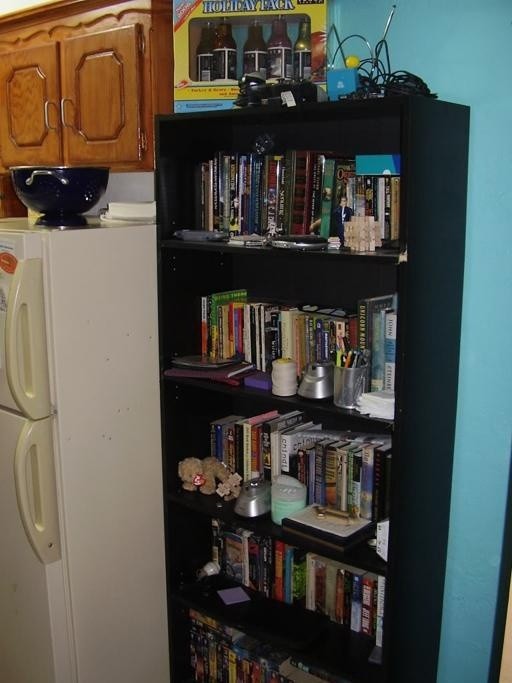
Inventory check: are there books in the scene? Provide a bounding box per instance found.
[210,411,391,550]
[165,294,396,419]
[208,522,386,648]
[190,610,334,683]
[200,153,401,242]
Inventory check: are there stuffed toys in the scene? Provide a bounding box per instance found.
[178,457,228,495]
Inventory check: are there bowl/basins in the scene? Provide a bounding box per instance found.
[9,167,111,228]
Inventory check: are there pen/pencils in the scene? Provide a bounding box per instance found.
[334,336,374,409]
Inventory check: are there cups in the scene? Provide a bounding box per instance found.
[332,363,370,409]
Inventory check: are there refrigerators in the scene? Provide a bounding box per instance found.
[1,212,170,681]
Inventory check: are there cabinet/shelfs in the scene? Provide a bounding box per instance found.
[151,97,471,682]
[1,21,146,169]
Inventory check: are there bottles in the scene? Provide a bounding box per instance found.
[194,14,313,87]
